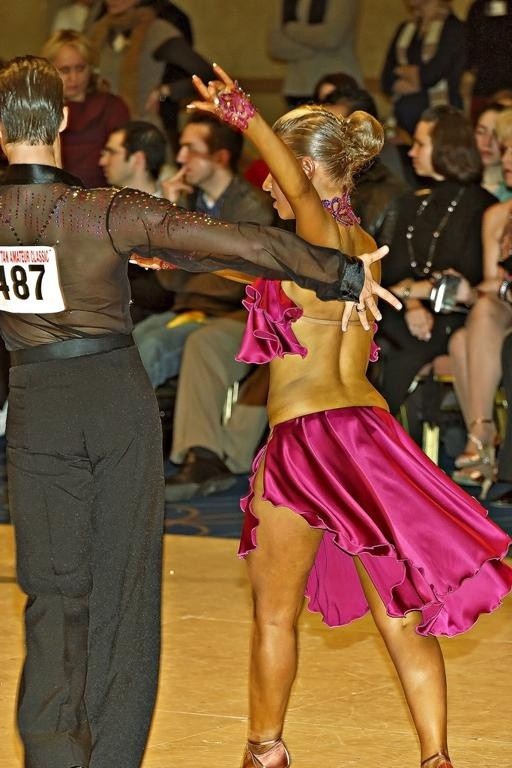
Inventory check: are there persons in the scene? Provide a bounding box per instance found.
[129,110,274,390]
[266,0,362,109]
[186,63,512,768]
[81,0,210,166]
[41,30,131,190]
[450,109,512,500]
[461,0,512,108]
[100,122,174,323]
[171,88,412,481]
[382,0,468,135]
[0,57,403,768]
[474,103,512,204]
[369,104,501,417]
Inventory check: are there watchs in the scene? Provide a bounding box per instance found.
[404,282,411,301]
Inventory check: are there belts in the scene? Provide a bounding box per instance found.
[3,331,141,369]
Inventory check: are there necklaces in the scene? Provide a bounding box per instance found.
[407,185,464,276]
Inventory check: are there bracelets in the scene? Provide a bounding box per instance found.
[499,279,510,301]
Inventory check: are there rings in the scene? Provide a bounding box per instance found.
[356,306,365,312]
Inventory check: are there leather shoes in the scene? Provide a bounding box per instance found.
[160,448,241,498]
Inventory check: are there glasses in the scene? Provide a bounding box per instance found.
[97,145,125,160]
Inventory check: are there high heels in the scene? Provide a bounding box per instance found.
[455,418,500,468]
[242,737,294,768]
[421,750,447,764]
[452,470,498,500]
